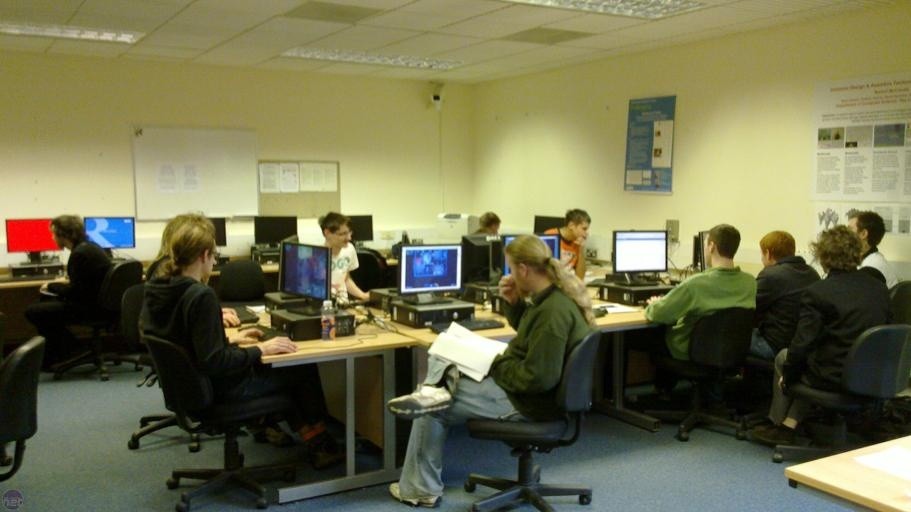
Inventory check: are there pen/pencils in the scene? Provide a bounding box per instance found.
[600,306,615,308]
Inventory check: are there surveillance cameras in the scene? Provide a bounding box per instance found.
[430,83,446,102]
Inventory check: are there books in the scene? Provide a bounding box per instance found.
[428,320,508,382]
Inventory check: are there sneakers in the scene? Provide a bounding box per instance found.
[389,482,441,508]
[387,384,453,420]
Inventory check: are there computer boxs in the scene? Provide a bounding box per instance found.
[390,296,474,328]
[370,288,402,311]
[599,285,677,306]
[491,294,504,315]
[449,284,500,304]
[264,292,308,314]
[271,307,354,341]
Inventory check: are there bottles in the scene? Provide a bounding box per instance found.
[318,299,337,339]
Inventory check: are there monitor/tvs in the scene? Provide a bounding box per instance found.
[207,217,227,247]
[83,216,136,249]
[276,235,304,298]
[398,244,462,305]
[347,215,373,241]
[6,219,65,253]
[461,215,709,286]
[254,216,298,245]
[280,241,338,315]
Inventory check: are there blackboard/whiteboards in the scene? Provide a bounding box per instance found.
[132,125,259,222]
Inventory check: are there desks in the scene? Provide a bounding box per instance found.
[346,286,662,441]
[579,253,700,298]
[781,431,911,511]
[213,294,418,507]
[1,249,399,293]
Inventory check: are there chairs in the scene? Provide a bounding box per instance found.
[2,332,49,485]
[121,283,162,394]
[649,305,758,443]
[49,258,145,387]
[465,327,600,511]
[139,336,291,511]
[887,278,910,324]
[771,324,911,467]
[220,259,267,302]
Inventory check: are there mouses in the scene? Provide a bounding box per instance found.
[597,308,608,314]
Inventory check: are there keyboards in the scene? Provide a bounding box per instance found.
[232,306,259,323]
[339,300,370,309]
[238,325,288,341]
[429,320,504,334]
[593,309,605,317]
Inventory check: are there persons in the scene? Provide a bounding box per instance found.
[386,233,599,508]
[475,211,502,235]
[644,212,901,450]
[544,210,592,279]
[26,215,113,375]
[319,213,371,306]
[142,214,346,449]
